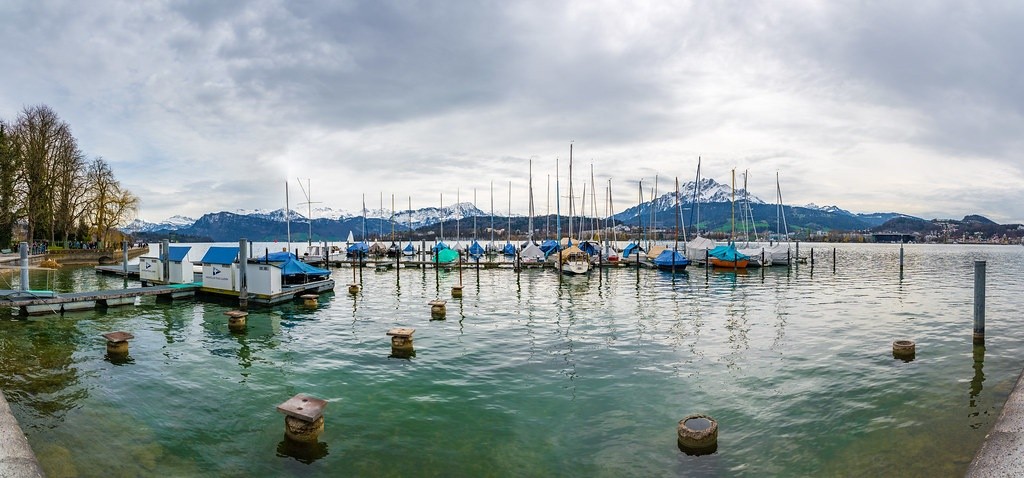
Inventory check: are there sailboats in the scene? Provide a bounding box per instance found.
[708,170,750,268]
[554,144,596,275]
[298,156,810,269]
